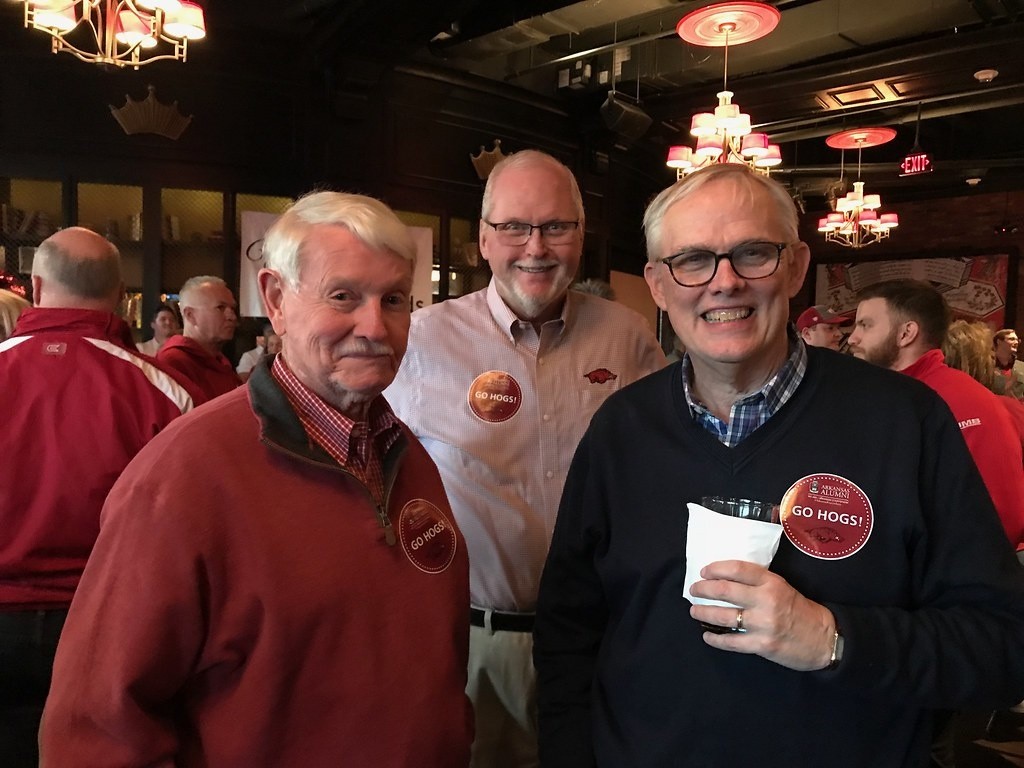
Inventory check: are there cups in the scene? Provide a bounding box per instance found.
[701,495,781,634]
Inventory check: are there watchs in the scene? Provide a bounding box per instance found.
[832,631,844,662]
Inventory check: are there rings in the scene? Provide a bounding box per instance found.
[737,609,743,629]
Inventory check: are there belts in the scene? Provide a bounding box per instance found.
[468,607,538,633]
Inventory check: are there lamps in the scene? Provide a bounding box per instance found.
[827,188,837,212]
[666,1,782,181]
[818,128,898,248]
[24,0,206,70]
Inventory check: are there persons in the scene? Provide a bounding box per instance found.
[39,193,471,768]
[992,329,1024,398]
[382,150,668,768]
[0,225,207,768]
[235,326,281,382]
[941,319,1017,399]
[667,335,686,363]
[847,278,1024,567]
[135,305,179,359]
[524,161,1024,768]
[154,275,242,399]
[796,305,849,351]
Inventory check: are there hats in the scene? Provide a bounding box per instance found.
[797,305,849,332]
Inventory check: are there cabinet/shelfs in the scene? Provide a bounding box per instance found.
[0,153,306,368]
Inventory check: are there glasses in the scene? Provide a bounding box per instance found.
[654,241,798,287]
[483,218,580,246]
